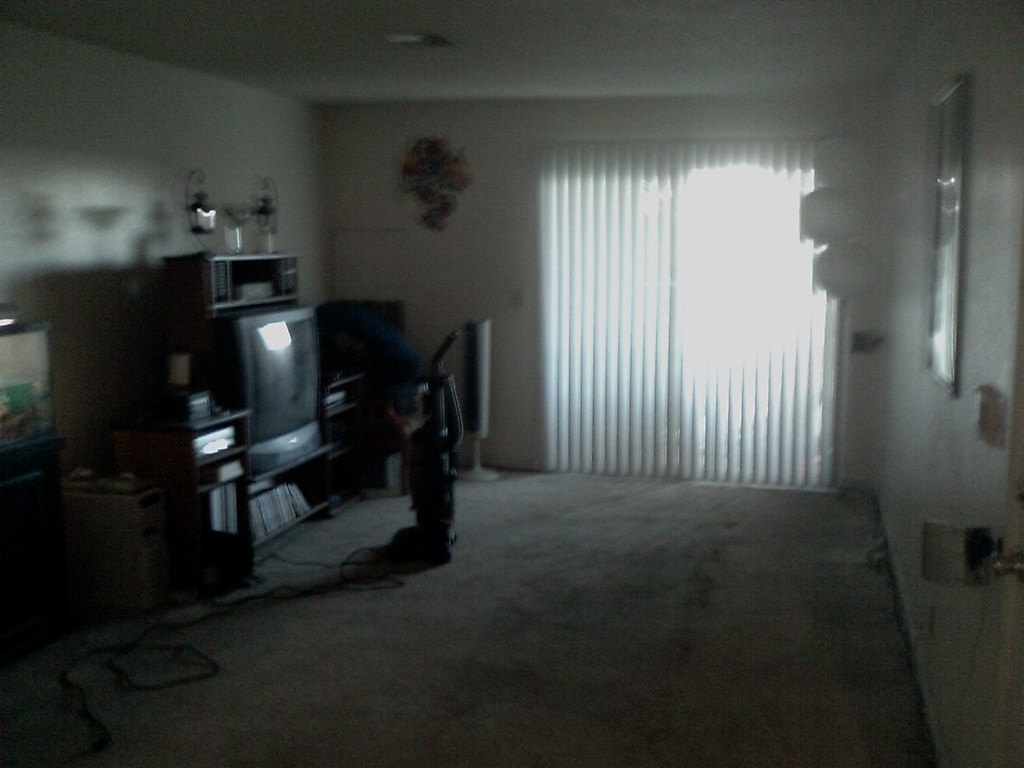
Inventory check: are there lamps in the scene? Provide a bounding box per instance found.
[256,178,282,254]
[184,169,215,256]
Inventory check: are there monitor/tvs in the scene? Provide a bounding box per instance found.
[194,302,322,476]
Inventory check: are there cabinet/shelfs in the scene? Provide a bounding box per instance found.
[0,432,75,668]
[112,254,377,590]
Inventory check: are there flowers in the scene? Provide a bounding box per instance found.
[403,135,475,231]
[221,203,255,227]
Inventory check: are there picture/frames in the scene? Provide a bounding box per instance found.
[922,75,971,397]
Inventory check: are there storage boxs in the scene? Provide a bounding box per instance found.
[0,318,55,449]
